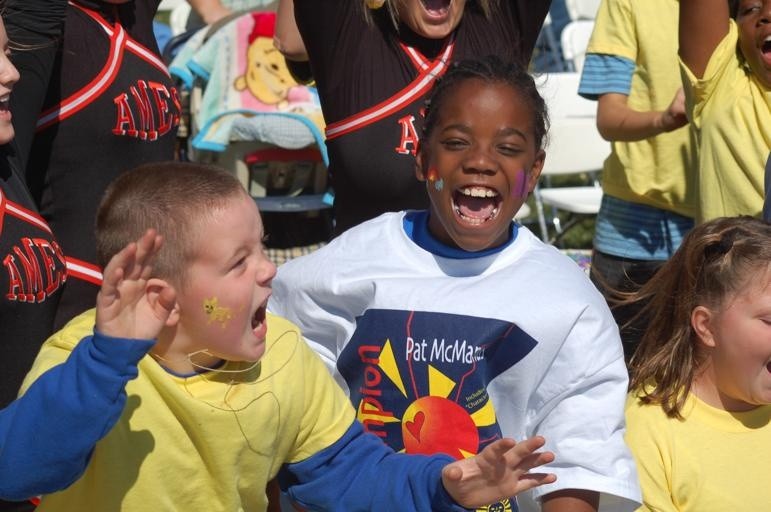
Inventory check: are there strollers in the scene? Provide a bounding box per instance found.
[165,4,338,244]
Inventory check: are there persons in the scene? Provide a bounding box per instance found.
[1,2,557,512]
[578,1,771,512]
[267,55,642,512]
